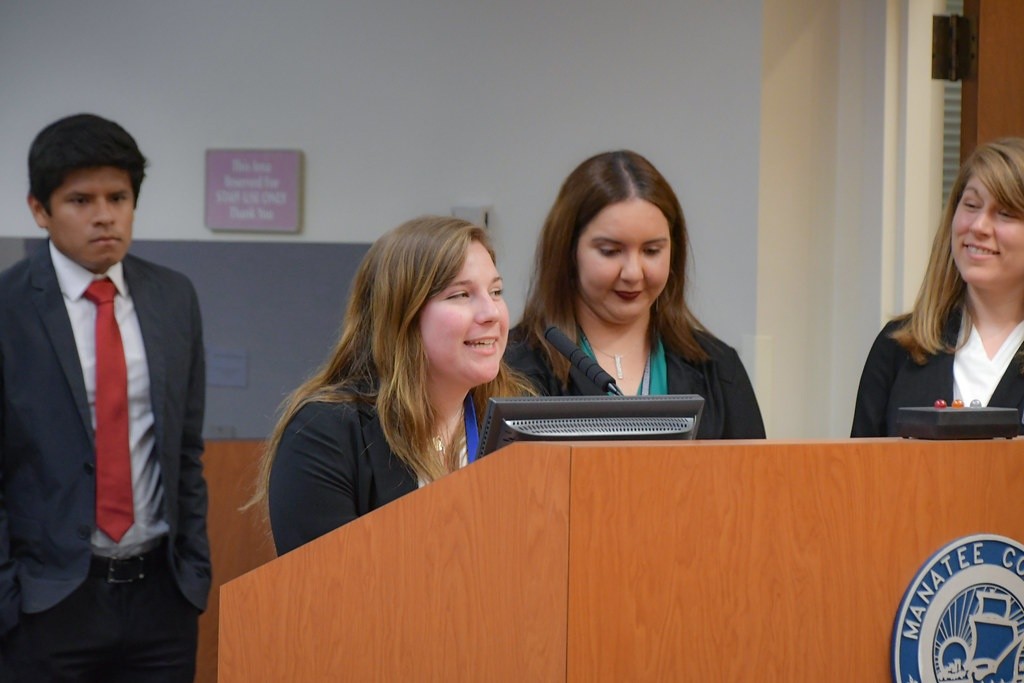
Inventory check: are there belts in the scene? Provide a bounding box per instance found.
[92,552,169,587]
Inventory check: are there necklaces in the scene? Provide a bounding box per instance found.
[583,330,652,380]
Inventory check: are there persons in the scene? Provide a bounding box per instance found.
[496,152,769,440]
[268,212,542,554]
[849,136,1024,436]
[0,110,211,683]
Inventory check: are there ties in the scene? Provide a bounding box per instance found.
[83,277,137,545]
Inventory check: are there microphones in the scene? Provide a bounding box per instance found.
[543,325,625,396]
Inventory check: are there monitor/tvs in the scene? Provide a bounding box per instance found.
[475,392,705,463]
